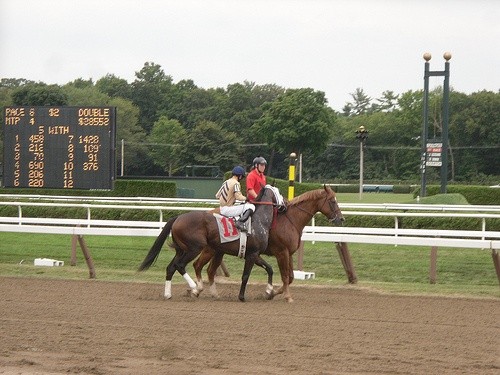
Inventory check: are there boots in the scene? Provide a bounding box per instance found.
[236,209,254,231]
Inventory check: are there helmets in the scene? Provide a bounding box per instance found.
[232,166,246,176]
[253,157,267,165]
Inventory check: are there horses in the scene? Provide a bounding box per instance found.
[192,184,346,303]
[135,179,288,304]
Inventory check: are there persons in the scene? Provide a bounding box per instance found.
[216,167,256,232]
[246,157,267,202]
